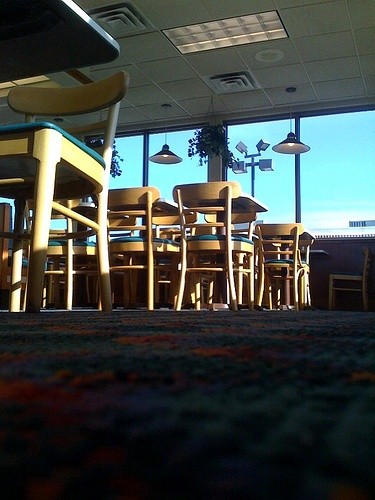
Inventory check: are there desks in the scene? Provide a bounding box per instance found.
[311,249,331,257]
[110,200,194,307]
[0,0,121,84]
[173,192,270,309]
[75,202,130,308]
[280,232,316,310]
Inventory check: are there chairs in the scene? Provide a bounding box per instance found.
[328,247,372,312]
[0,69,314,314]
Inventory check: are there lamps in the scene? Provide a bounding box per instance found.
[272,86,311,154]
[148,103,183,165]
